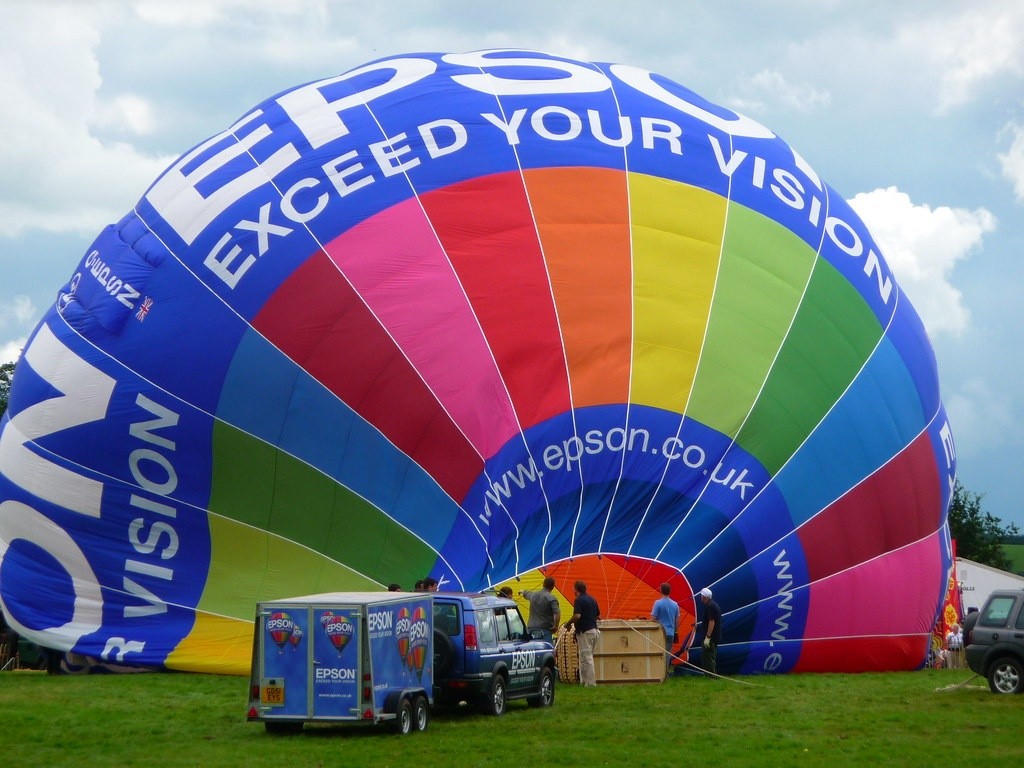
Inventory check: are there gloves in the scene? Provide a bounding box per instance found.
[703,635,711,649]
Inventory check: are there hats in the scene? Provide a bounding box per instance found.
[700,588,712,599]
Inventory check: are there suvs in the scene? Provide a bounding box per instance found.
[962,587,1024,695]
[427,591,559,717]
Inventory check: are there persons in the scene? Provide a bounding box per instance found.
[422,578,437,592]
[499,586,514,599]
[564,581,601,686]
[518,577,561,679]
[945,625,964,668]
[415,580,425,592]
[698,588,720,679]
[650,583,679,670]
[388,584,401,592]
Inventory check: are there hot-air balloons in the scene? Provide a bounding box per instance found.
[287,624,303,654]
[327,615,354,659]
[1,51,971,680]
[268,611,294,654]
[410,607,428,686]
[405,633,415,683]
[395,607,411,676]
[320,610,334,631]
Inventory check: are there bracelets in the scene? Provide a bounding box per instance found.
[675,632,678,633]
[567,623,571,627]
[706,634,711,638]
